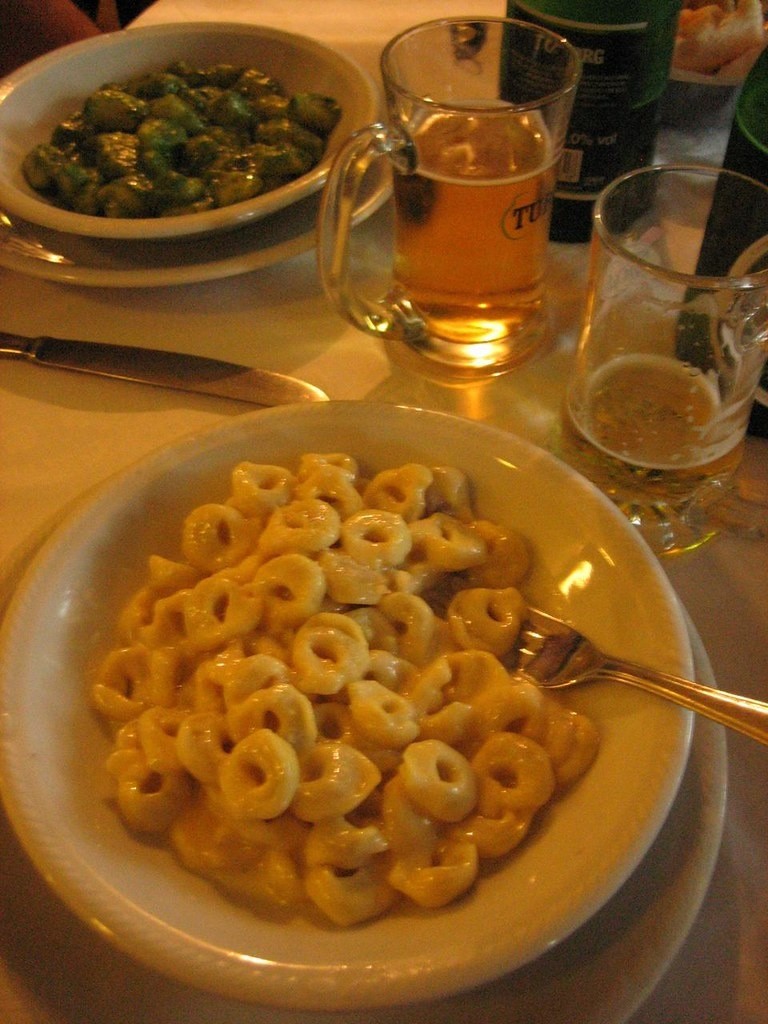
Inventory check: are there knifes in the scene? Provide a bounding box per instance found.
[1,328,332,415]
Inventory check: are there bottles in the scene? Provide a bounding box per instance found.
[672,43,768,447]
[496,0,679,245]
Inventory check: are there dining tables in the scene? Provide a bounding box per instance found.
[1,0,768,1024]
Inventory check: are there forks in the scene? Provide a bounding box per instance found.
[425,574,768,756]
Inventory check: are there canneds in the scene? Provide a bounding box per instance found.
[498,0,681,244]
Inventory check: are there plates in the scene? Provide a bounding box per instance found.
[0,402,726,1024]
[0,23,392,286]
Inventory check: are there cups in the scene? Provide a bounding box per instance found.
[554,165,768,563]
[313,15,580,378]
